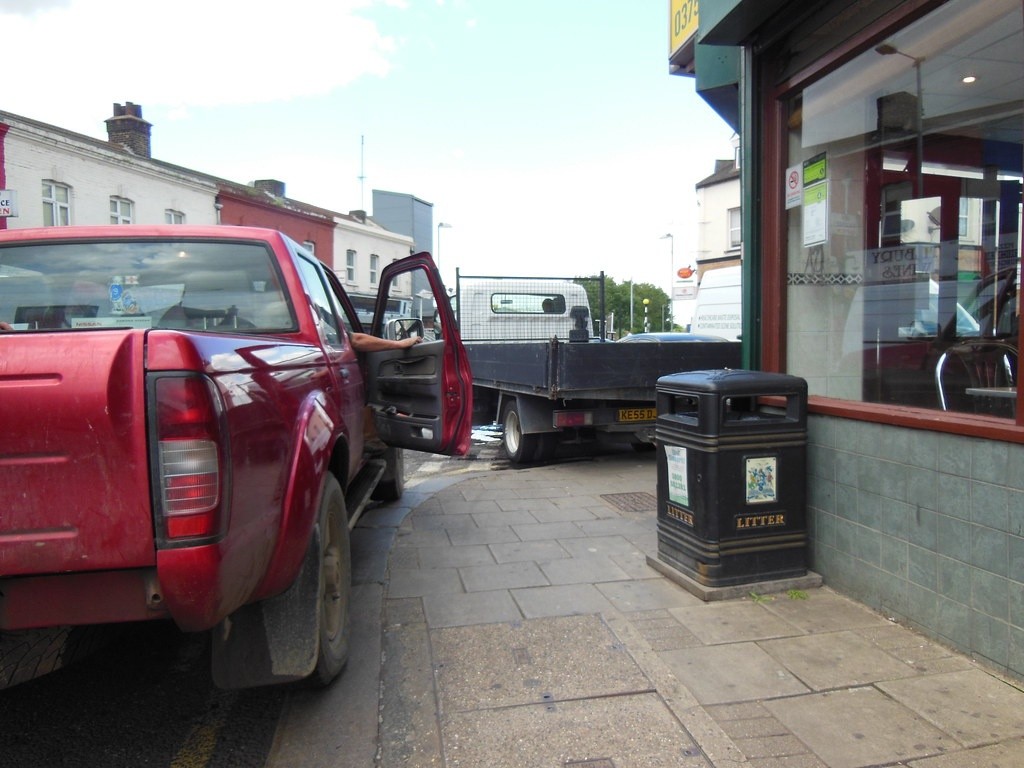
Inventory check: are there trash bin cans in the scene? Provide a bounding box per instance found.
[655,371,809,587]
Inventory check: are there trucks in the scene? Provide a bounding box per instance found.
[431,267,743,467]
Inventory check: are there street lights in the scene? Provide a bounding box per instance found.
[659,234,674,332]
[437,223,453,274]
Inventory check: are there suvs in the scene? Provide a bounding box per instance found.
[0,223,475,703]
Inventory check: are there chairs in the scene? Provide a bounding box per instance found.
[934,338,1019,413]
[181,273,239,330]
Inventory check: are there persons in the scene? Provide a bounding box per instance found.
[258,279,424,454]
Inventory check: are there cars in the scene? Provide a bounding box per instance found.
[613,332,730,342]
